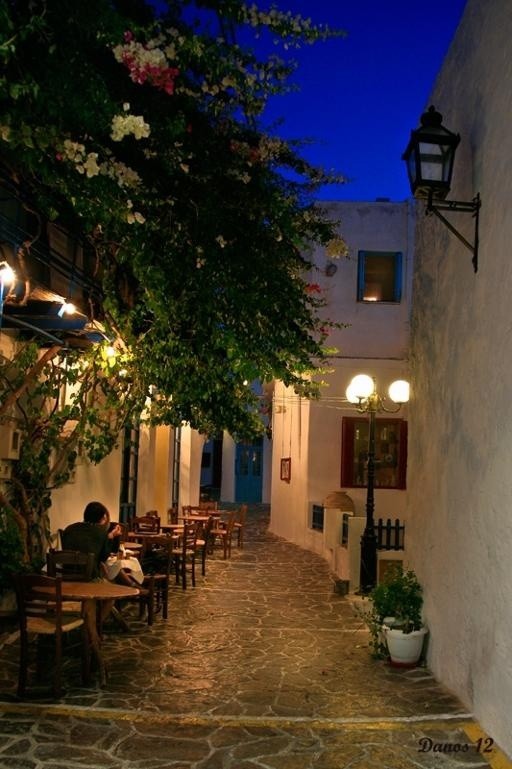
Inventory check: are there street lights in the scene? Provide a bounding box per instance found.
[346,374,409,594]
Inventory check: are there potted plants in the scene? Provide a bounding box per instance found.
[362,558,429,668]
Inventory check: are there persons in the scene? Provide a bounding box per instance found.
[102,505,152,628]
[61,500,123,643]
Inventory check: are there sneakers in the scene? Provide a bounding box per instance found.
[125,584,149,598]
[99,623,124,633]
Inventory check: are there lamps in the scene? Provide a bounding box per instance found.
[403,104,481,274]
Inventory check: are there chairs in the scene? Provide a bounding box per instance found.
[0,494,249,699]
